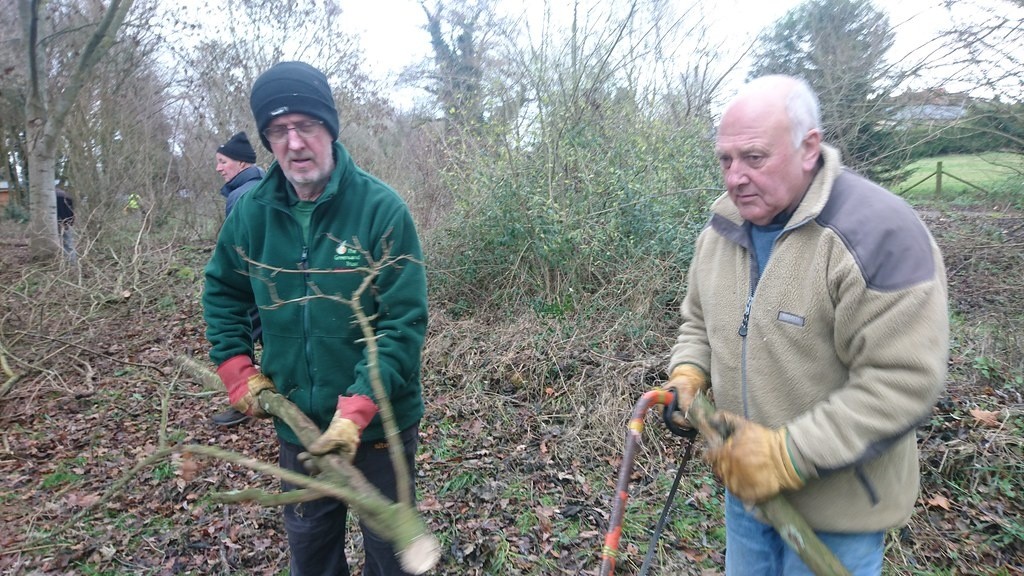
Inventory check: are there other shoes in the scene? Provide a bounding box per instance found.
[211,408,249,425]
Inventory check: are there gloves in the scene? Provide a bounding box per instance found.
[700,409,809,503]
[651,364,710,428]
[297,393,379,472]
[216,355,277,420]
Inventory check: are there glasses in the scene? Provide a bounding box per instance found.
[262,119,327,144]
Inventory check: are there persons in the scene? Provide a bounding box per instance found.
[203,59,430,576]
[661,73,950,576]
[127,192,141,209]
[215,133,264,425]
[57,187,77,265]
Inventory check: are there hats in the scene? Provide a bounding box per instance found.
[249,62,339,154]
[216,131,257,163]
